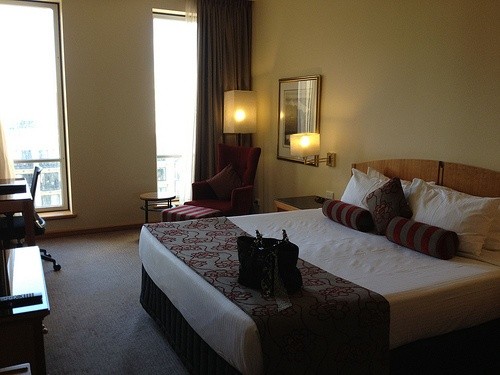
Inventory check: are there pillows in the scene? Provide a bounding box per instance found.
[321,168,500,261]
[205,163,247,201]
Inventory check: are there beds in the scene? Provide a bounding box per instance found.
[138,159,500,375]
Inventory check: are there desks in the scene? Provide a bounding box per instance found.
[0,178,37,247]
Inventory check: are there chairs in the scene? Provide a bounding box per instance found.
[0,166,61,272]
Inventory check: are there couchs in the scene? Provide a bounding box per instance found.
[184,142,261,216]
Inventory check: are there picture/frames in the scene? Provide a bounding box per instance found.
[276,75,323,169]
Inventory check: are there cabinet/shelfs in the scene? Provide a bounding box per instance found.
[0,246,52,375]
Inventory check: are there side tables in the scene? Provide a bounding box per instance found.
[139,190,176,223]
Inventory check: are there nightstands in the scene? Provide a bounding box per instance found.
[272,195,332,214]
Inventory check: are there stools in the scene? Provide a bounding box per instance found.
[160,204,222,222]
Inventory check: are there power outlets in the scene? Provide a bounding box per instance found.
[326,191,334,200]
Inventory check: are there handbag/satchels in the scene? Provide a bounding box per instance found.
[236,229,304,299]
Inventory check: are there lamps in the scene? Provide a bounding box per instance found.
[222,89,257,146]
[289,133,337,168]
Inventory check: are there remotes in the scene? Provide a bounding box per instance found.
[0,292,43,308]
